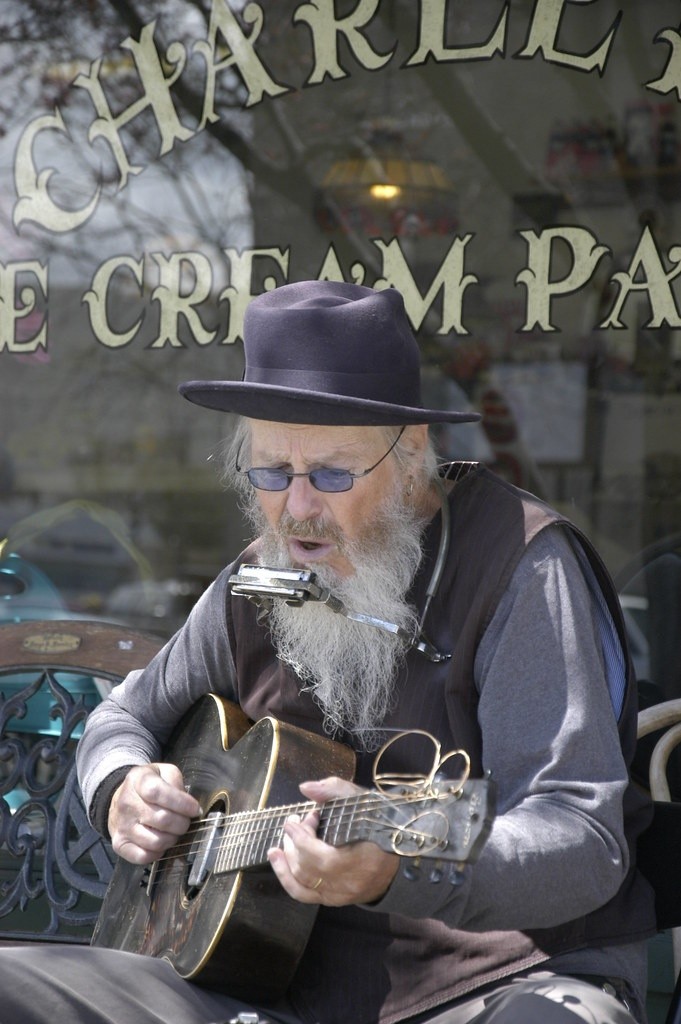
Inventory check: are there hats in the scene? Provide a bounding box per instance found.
[178,280,482,427]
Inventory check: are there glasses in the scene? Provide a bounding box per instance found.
[236,424,407,493]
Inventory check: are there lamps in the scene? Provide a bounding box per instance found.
[315,0,459,233]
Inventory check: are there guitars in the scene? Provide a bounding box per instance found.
[88,691,498,1004]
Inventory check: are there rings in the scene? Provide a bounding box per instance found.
[313,877,323,890]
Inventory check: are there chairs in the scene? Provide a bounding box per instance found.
[2,612,680,1023]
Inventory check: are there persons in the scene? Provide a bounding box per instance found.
[1,276,681,1023]
